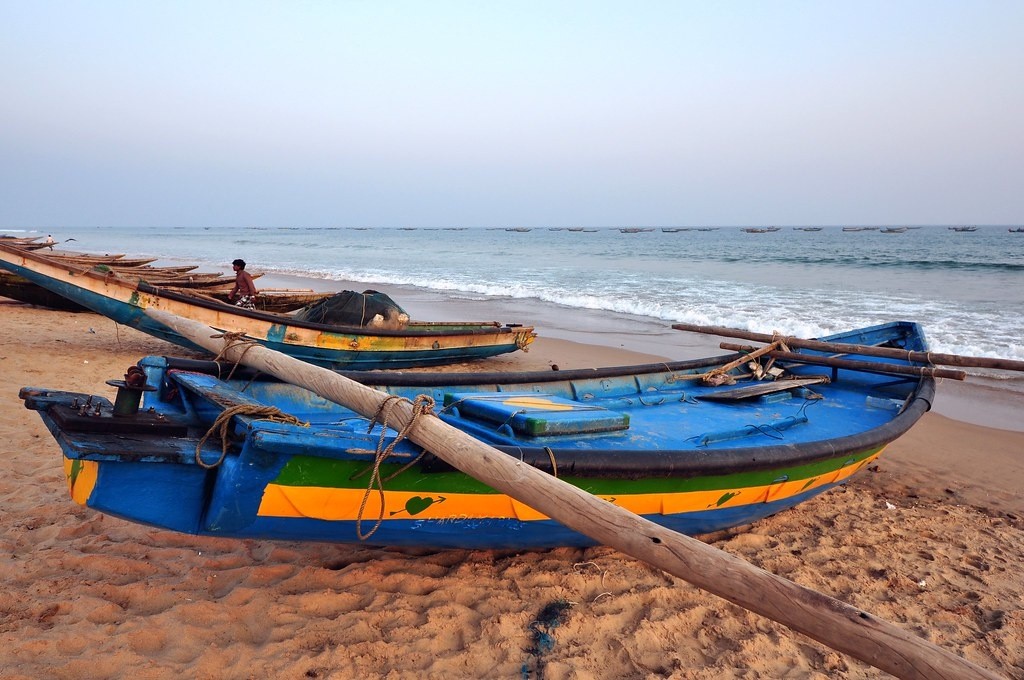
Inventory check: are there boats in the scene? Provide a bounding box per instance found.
[15,321,936,549]
[618,225,723,234]
[546,226,604,232]
[792,225,824,232]
[739,225,783,233]
[485,225,543,232]
[841,225,924,233]
[948,225,981,231]
[0,235,539,372]
[1008,226,1024,232]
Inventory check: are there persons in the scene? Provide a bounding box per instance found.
[228,259,257,307]
[47,235,55,251]
